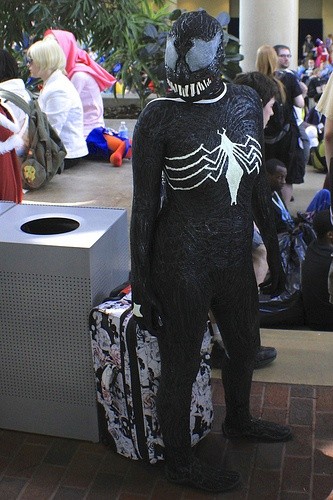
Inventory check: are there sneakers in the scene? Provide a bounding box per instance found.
[214,340,277,369]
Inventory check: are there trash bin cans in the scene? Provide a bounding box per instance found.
[0,203,131,441]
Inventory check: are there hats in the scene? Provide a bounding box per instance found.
[223,38,244,63]
[306,35,312,38]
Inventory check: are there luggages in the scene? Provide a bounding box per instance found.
[89,282,213,465]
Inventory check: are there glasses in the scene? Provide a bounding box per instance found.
[278,54,292,58]
[26,56,34,65]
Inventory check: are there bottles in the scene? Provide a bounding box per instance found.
[119,121,129,138]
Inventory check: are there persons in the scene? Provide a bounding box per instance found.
[199,33,333,368]
[0,29,115,203]
[128,11,293,493]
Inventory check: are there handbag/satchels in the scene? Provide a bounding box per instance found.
[83,125,131,160]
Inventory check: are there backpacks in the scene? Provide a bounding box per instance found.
[0,88,67,191]
